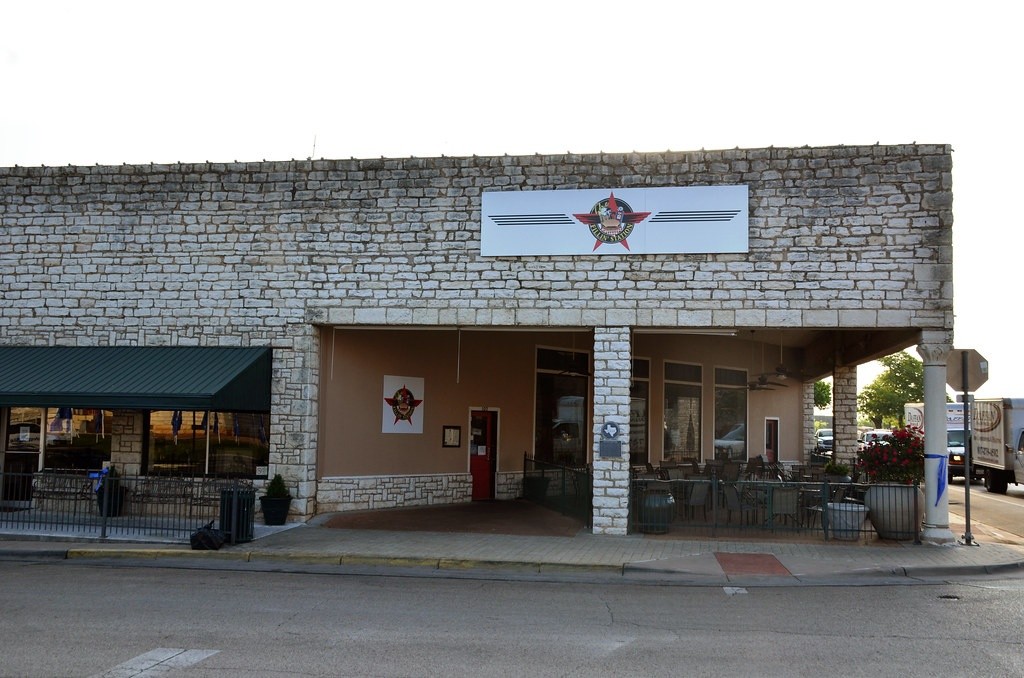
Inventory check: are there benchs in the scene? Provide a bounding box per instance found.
[34,469,93,513]
[196,477,252,518]
[131,477,193,519]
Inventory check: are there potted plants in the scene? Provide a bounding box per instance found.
[97,466,122,517]
[258,474,292,525]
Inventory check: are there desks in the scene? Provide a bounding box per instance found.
[787,468,824,480]
[748,476,789,527]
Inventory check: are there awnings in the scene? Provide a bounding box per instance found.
[0,346,271,475]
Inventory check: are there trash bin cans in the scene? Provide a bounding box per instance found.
[218,487,259,543]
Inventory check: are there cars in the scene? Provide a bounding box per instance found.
[9,422,59,446]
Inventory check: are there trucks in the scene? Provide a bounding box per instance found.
[972,397,1024,494]
[552,396,646,454]
[904,402,982,483]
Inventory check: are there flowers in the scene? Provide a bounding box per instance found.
[856,425,925,485]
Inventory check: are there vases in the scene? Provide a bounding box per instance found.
[827,503,870,541]
[864,482,926,539]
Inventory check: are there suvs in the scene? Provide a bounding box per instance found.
[814,429,833,454]
[856,432,894,451]
[715,423,745,458]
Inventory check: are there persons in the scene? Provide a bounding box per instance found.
[94,463,118,517]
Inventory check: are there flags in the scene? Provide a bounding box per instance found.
[50,408,102,434]
[171,410,266,445]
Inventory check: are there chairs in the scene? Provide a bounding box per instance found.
[586,454,825,533]
